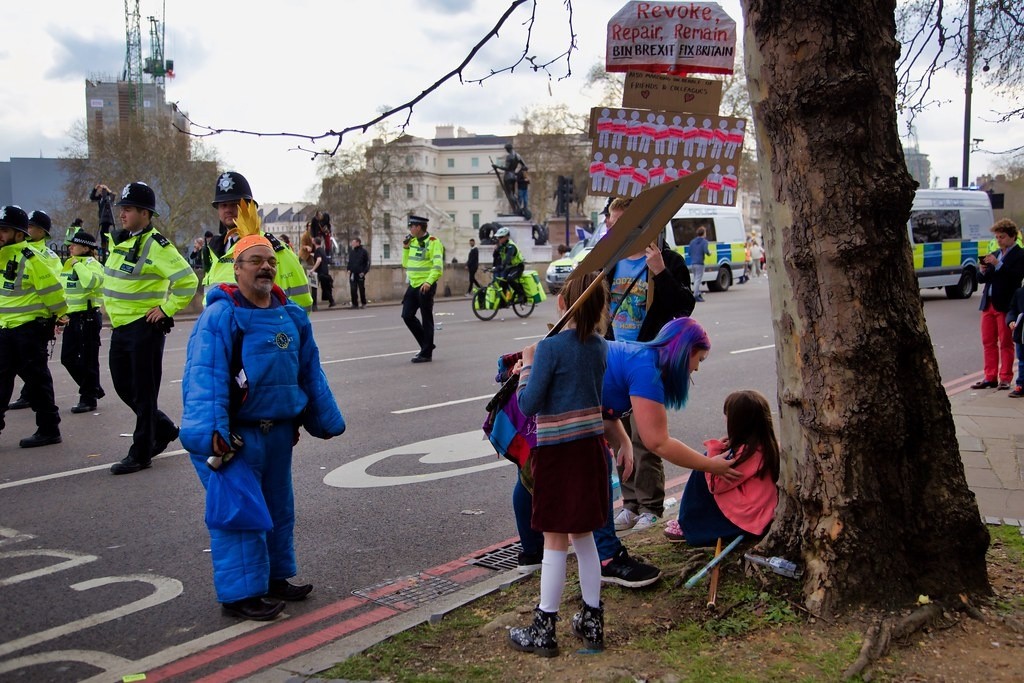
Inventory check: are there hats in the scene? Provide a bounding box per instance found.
[233,235,276,261]
[407,215,429,227]
[211,172,260,209]
[598,196,616,215]
[114,182,161,217]
[67,233,98,248]
[27,210,52,239]
[0,206,31,237]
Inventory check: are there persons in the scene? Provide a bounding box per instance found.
[517,166,531,209]
[970,218,1024,398]
[507,195,782,659]
[401,214,444,363]
[347,238,370,309]
[689,226,713,303]
[0,172,346,621]
[492,144,529,216]
[737,229,765,286]
[490,227,528,304]
[464,239,482,297]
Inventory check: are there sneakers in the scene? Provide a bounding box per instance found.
[696,296,704,302]
[599,545,663,588]
[613,508,640,530]
[0,345,436,474]
[517,551,544,573]
[1008,386,1024,398]
[663,527,685,540]
[666,520,681,528]
[631,512,660,532]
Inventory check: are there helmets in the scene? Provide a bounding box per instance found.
[494,227,510,238]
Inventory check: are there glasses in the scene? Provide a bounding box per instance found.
[239,256,277,267]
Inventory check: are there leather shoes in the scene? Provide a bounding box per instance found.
[268,579,313,601]
[997,382,1010,390]
[971,379,998,389]
[222,597,287,621]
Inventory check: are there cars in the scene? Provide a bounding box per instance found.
[545,238,592,295]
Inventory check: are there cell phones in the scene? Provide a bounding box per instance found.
[979,256,990,266]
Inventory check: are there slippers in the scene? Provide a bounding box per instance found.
[508,603,562,658]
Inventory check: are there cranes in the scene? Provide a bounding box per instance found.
[118,0,174,131]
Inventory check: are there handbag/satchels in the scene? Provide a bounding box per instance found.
[331,237,340,254]
[307,270,319,289]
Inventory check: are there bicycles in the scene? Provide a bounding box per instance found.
[471,266,536,320]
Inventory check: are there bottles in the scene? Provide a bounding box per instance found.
[506,291,511,299]
[205,433,244,471]
[744,552,804,579]
[663,497,678,509]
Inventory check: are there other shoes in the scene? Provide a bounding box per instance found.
[312,293,471,312]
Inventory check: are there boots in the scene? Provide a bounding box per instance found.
[572,600,605,651]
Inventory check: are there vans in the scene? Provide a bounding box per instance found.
[572,202,747,292]
[905,187,997,298]
